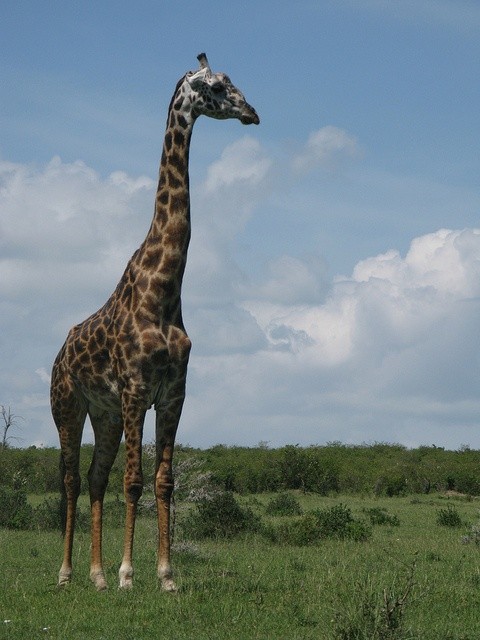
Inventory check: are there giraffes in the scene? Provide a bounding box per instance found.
[50,52,260,592]
